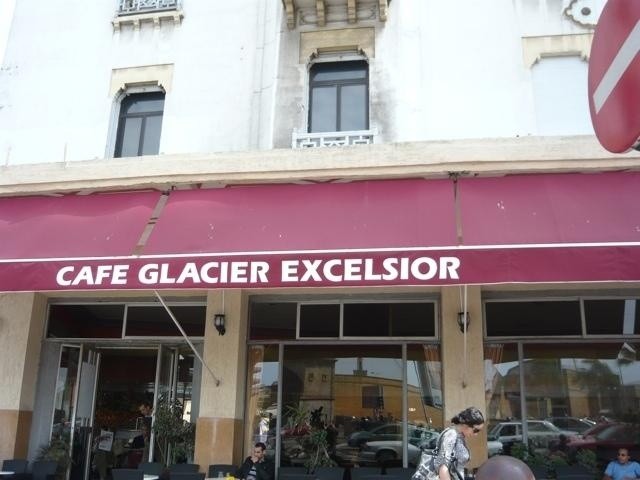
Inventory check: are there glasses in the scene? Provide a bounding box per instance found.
[470,424,480,434]
[617,454,629,457]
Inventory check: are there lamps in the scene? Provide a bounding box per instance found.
[214,314,225,335]
[457,311,471,333]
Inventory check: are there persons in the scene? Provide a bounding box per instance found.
[234,442,274,480]
[435,406,485,480]
[92,402,152,479]
[604,445,640,480]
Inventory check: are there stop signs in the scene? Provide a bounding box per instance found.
[589,0,640,153]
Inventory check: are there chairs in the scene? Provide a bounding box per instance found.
[533,464,608,480]
[111,462,416,480]
[0,458,63,480]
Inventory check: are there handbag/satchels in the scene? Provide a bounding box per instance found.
[412,449,457,480]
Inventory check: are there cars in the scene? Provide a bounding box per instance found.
[542,420,640,466]
[361,427,442,468]
[280,405,640,472]
